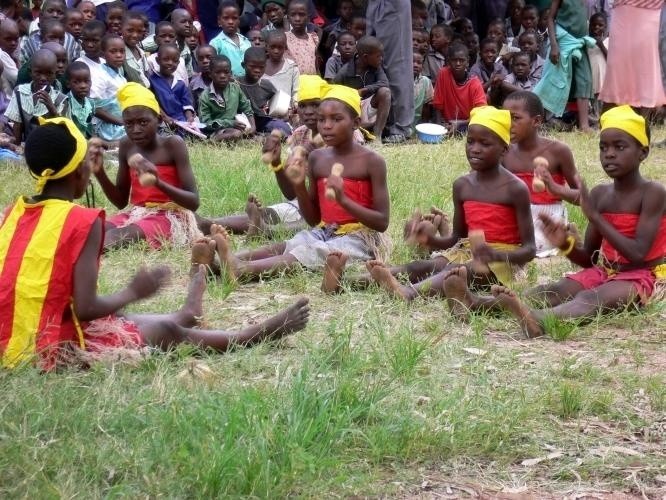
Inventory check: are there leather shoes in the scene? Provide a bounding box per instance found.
[382,135,408,143]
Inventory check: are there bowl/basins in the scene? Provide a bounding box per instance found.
[415,123,448,143]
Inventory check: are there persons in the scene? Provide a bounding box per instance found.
[187,84,391,283]
[76,80,201,256]
[441,102,666,340]
[319,103,537,303]
[0,0,666,165]
[404,89,583,260]
[0,116,312,372]
[192,73,329,243]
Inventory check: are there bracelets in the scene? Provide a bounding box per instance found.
[557,235,576,258]
[267,160,285,172]
